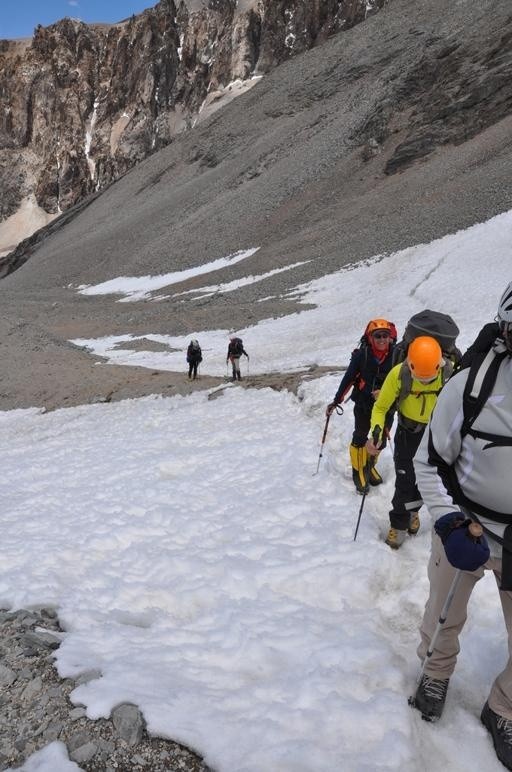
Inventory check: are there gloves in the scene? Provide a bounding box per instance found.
[434,512,489,571]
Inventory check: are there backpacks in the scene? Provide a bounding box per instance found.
[392,309,462,405]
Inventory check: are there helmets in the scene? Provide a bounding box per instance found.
[407,336,442,382]
[498,281,512,355]
[368,319,391,336]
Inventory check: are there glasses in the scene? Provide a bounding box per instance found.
[374,333,388,338]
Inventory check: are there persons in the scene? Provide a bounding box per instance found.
[408,276,511,772]
[223,336,252,381]
[185,338,203,381]
[361,335,458,552]
[323,319,399,495]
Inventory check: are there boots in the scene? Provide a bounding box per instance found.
[350,444,382,494]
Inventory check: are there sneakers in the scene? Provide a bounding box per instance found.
[385,511,420,550]
[416,673,449,720]
[481,700,512,771]
